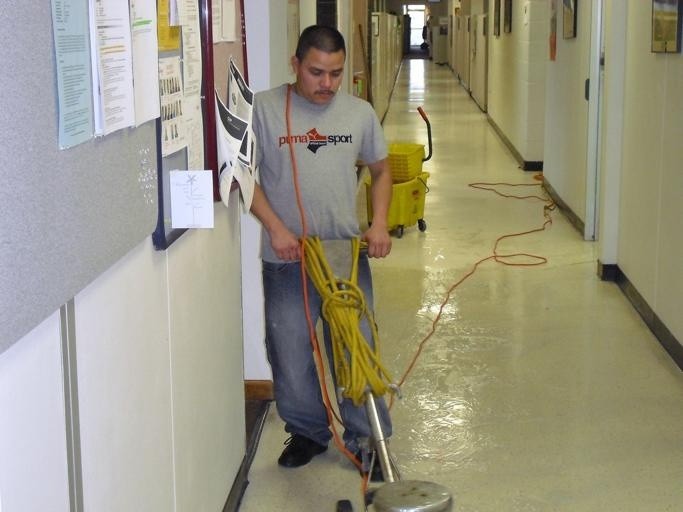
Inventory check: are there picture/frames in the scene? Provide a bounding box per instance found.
[199,0,251,204]
[493,1,682,54]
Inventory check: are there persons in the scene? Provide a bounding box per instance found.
[238,23,392,482]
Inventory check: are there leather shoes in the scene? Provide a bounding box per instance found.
[278,433,328,467]
[345,438,382,481]
